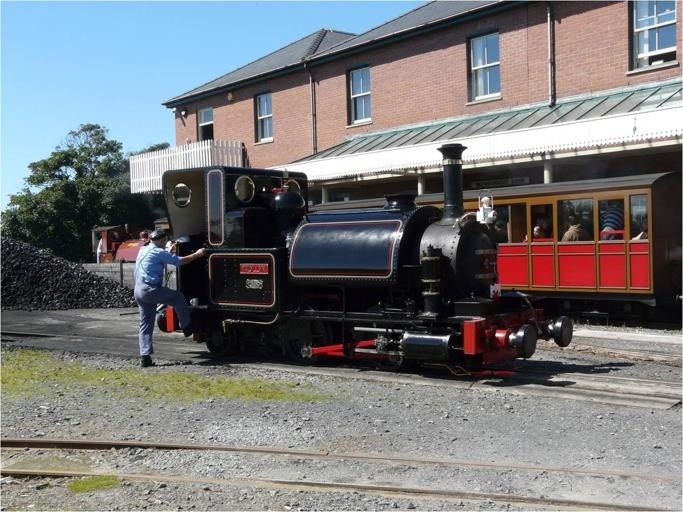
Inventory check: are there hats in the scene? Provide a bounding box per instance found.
[149,230,166,241]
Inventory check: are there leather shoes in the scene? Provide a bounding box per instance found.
[140,355,151,367]
[183,326,195,337]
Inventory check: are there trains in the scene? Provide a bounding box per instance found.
[414,169,682,317]
[152,142,573,380]
[89,223,170,265]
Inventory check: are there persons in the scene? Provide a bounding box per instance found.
[132,229,208,368]
[639,217,648,239]
[183,137,191,144]
[598,200,625,239]
[521,226,546,240]
